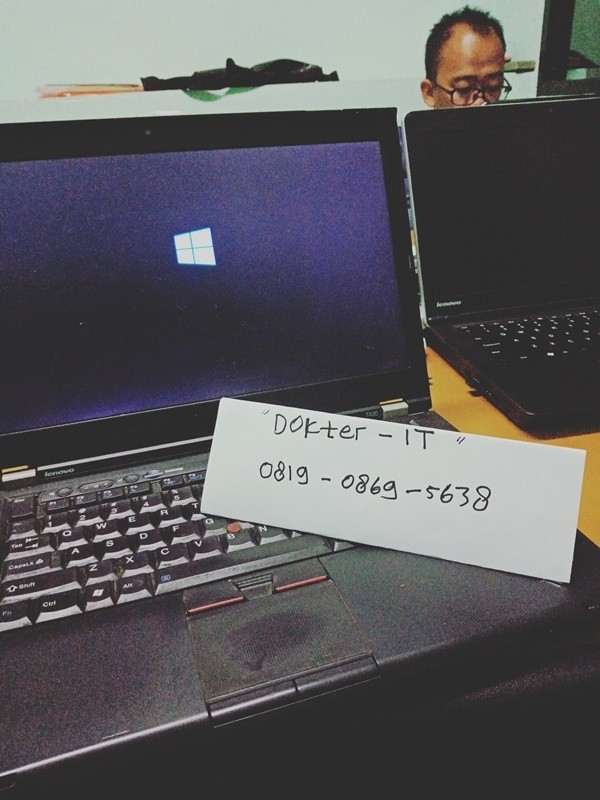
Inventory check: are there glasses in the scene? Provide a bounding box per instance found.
[434,76,512,105]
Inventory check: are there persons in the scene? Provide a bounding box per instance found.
[398,6,512,273]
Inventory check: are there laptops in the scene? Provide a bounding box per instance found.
[403,95,600,440]
[0,107,600,800]
